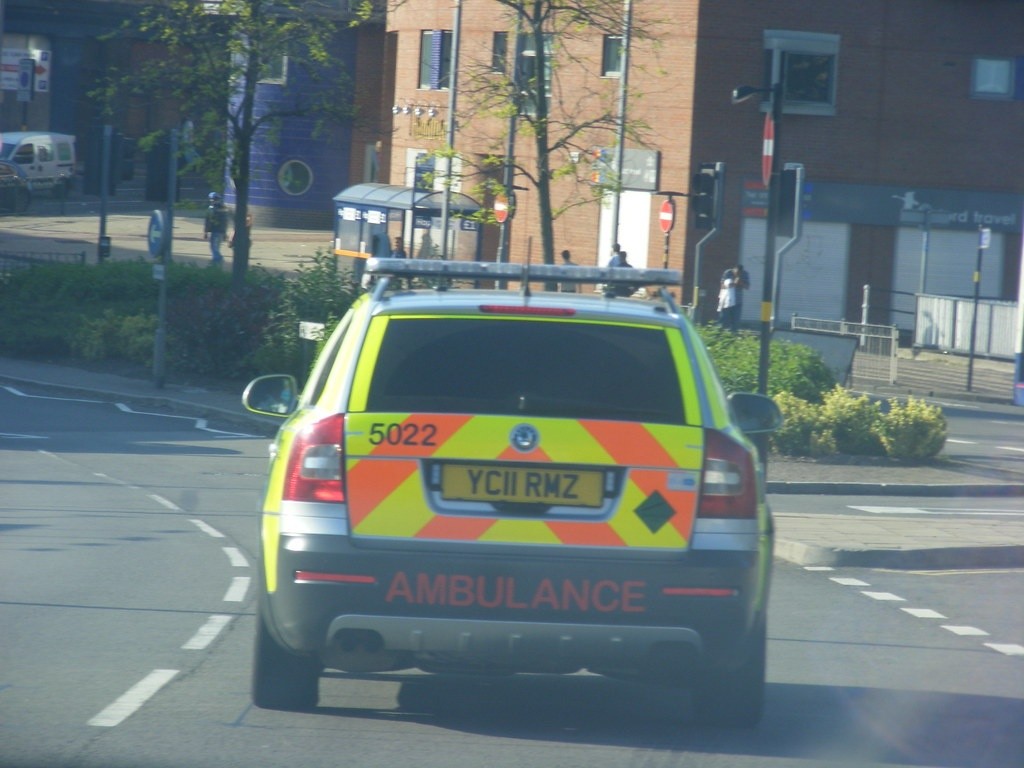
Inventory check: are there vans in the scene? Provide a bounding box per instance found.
[0,131,76,181]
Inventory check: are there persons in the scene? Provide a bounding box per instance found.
[204,190,228,265]
[601,242,641,296]
[557,249,584,293]
[390,236,407,260]
[716,259,750,333]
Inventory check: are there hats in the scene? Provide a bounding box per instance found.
[207,191,222,199]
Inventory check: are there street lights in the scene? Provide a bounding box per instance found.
[729,48,789,481]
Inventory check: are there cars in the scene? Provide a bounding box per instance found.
[241,256,785,731]
